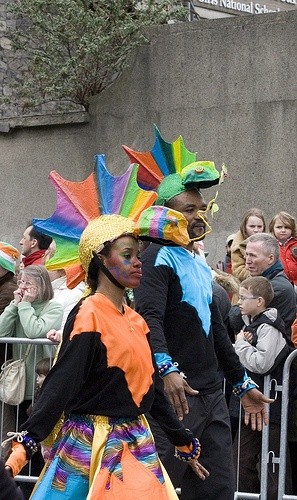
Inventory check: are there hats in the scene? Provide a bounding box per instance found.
[30,154,194,290]
[0,240,20,276]
[118,123,225,207]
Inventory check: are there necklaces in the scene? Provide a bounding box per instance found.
[118,309,133,332]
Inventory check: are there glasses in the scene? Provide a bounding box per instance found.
[17,279,33,288]
[237,295,257,301]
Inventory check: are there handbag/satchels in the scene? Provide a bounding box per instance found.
[0,358,27,407]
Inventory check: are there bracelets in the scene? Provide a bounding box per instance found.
[173,437,202,464]
[231,378,260,397]
[157,360,178,375]
[1,431,39,456]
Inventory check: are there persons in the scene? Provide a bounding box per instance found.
[122,123,274,500]
[0,207,297,500]
[2,154,210,500]
